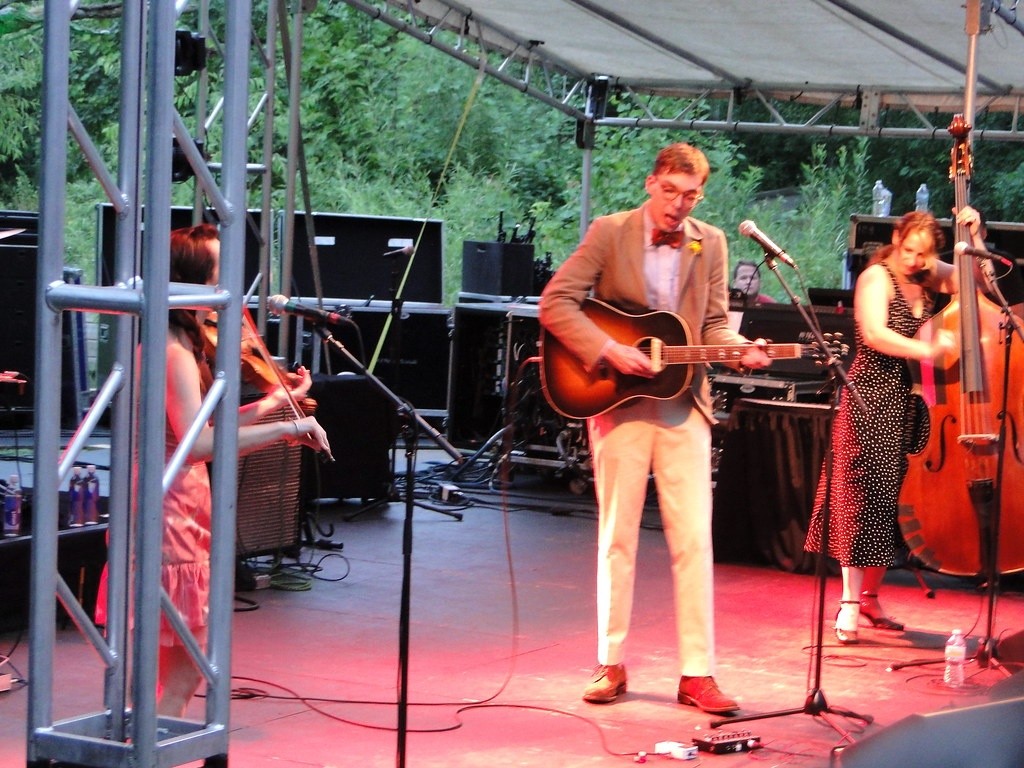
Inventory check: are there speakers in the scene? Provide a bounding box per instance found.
[461,240,534,296]
[233,389,308,561]
[840,670,1024,768]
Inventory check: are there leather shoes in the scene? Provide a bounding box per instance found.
[677,675,741,712]
[582,662,628,702]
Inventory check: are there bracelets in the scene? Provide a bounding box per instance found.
[287,417,300,438]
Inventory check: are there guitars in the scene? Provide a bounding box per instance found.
[537,295,849,420]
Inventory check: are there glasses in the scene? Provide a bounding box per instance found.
[654,176,704,204]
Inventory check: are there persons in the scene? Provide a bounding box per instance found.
[731,259,777,303]
[803,205,998,645]
[93,221,333,720]
[538,142,773,711]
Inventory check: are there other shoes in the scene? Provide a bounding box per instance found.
[835,600,860,642]
[859,592,904,631]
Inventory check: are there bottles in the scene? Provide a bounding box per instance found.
[67,467,84,527]
[916,183,929,212]
[3,475,22,536]
[943,629,966,687]
[83,465,99,526]
[872,179,892,217]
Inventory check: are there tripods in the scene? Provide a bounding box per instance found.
[891,258,1024,679]
[342,431,464,521]
[709,256,875,745]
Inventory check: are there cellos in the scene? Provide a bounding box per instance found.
[897,113,1024,667]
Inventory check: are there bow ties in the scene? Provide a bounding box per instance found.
[651,228,684,249]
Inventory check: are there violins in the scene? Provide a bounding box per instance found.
[195,308,330,463]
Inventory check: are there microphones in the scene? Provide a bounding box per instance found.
[953,241,1013,267]
[383,246,415,257]
[269,295,351,326]
[738,220,799,272]
